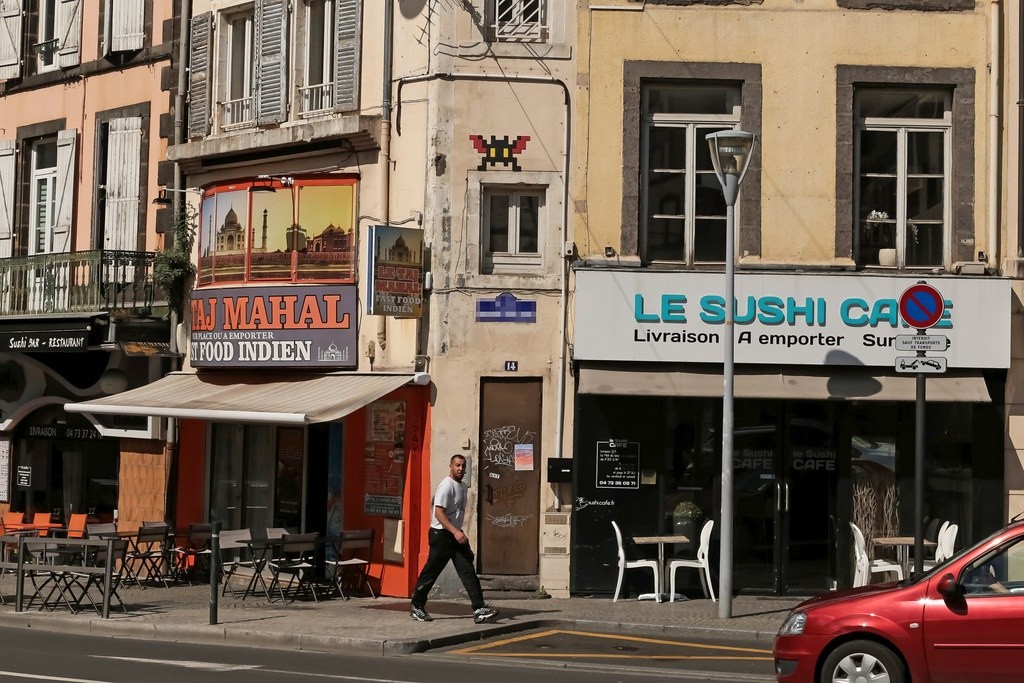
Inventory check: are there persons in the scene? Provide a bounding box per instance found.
[987,581,1024,596]
[409,454,501,625]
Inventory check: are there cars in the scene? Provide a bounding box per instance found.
[772,512,1024,682]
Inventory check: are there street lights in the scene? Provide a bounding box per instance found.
[705,128,758,624]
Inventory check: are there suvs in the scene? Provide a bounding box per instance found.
[703,425,896,493]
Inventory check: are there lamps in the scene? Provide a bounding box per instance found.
[152,188,206,205]
[248,174,294,193]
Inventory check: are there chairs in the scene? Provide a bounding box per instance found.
[849,521,904,588]
[907,524,958,580]
[664,517,717,603]
[849,524,897,588]
[907,520,950,580]
[611,520,661,603]
[0,500,374,615]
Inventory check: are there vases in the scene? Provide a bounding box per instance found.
[877,248,896,267]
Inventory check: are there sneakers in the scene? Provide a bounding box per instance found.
[474,606,499,624]
[410,604,432,621]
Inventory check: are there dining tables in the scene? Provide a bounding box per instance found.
[632,536,690,601]
[870,536,938,580]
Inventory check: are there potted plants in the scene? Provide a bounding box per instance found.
[664,500,703,587]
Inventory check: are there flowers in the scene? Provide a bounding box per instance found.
[864,209,920,248]
